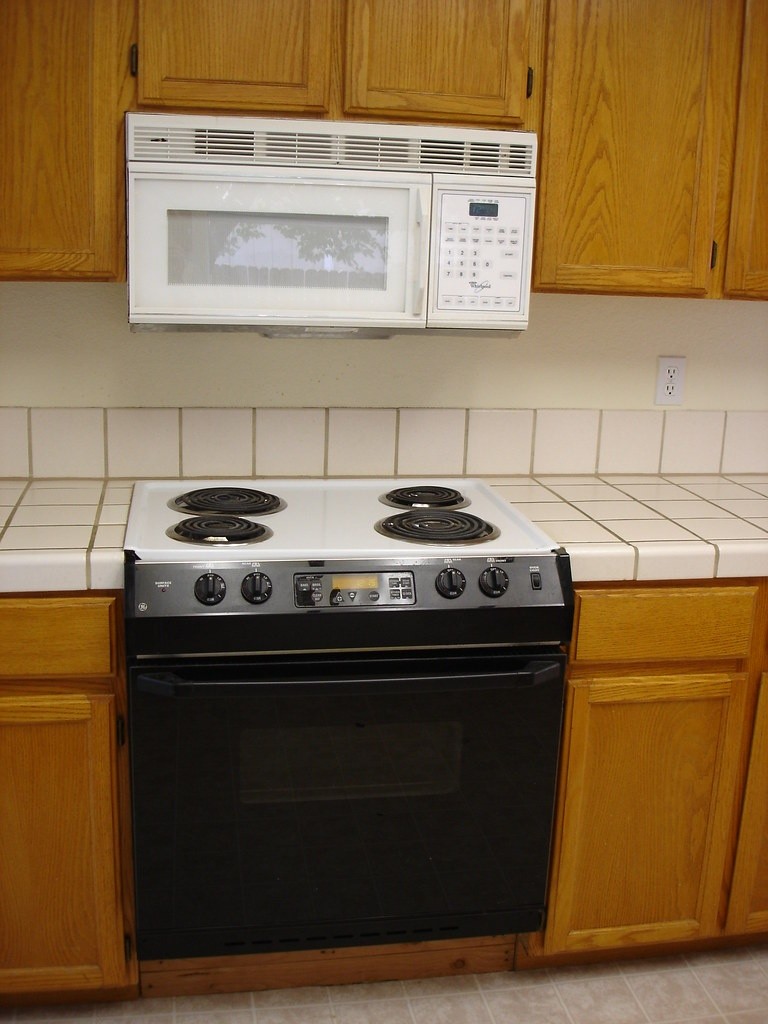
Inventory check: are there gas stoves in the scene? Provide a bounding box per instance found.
[124,478,564,654]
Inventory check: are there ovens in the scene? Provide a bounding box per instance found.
[128,661,566,961]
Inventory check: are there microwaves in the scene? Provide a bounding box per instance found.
[123,112,538,340]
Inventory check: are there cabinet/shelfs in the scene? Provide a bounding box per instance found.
[0,591,140,1003]
[542,586,767,954]
[1,0,767,299]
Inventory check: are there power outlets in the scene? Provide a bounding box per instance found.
[652,356,684,406]
